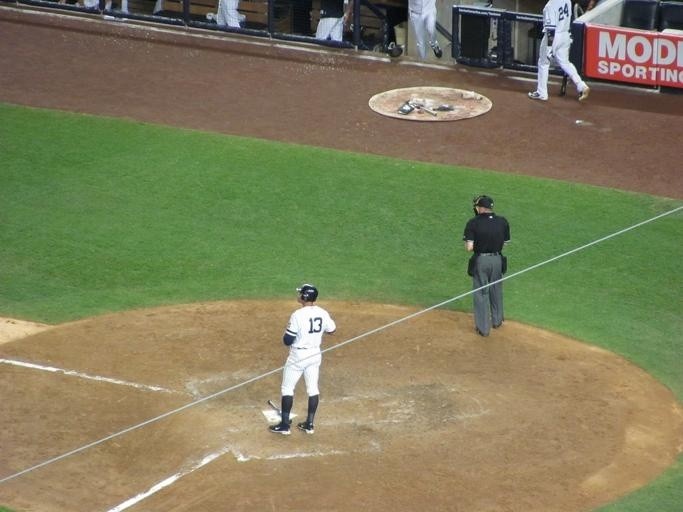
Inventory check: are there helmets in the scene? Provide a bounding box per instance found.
[296,285,318,301]
[474,196,494,214]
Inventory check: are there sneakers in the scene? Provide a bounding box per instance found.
[433,45,442,57]
[528,91,549,101]
[269,422,291,434]
[297,422,315,434]
[579,86,590,102]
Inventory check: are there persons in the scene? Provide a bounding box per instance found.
[407,0,442,60]
[527,0,590,102]
[316,0,354,43]
[216,0,241,29]
[463,194,511,337]
[268,283,336,436]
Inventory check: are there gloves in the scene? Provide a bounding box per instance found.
[569,32,574,43]
[547,46,554,62]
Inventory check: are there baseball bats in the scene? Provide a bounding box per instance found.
[560,44,570,95]
[422,106,438,116]
[268,400,292,424]
